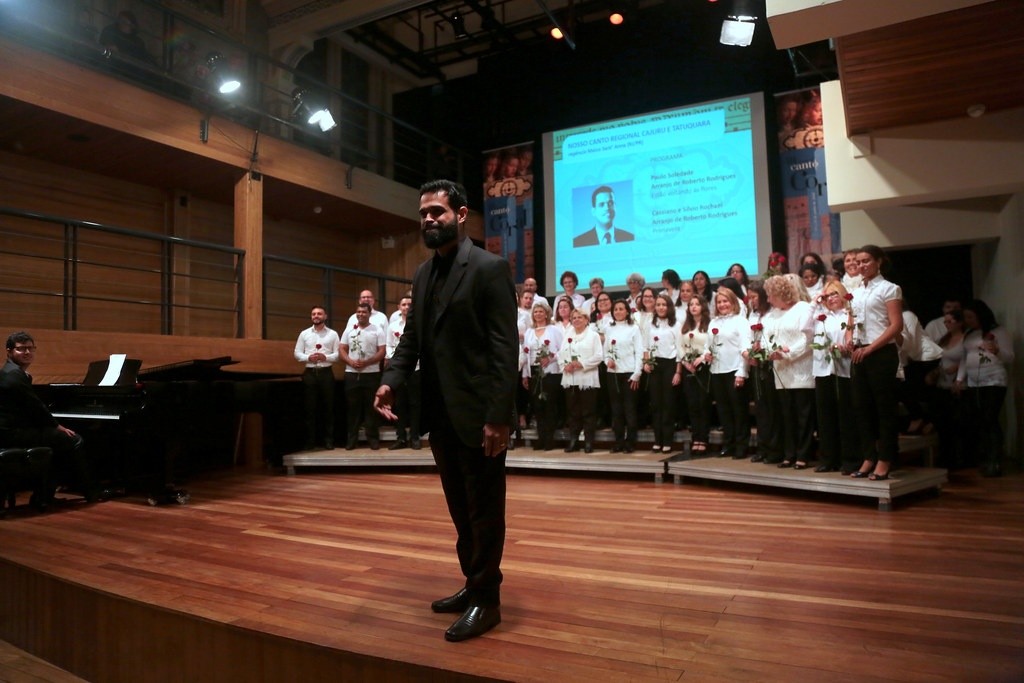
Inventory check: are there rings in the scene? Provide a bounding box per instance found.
[500,443,505,447]
[835,345,838,348]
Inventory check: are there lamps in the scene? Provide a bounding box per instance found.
[550,9,567,39]
[286,89,336,131]
[719,0,764,47]
[610,0,640,25]
[196,53,242,95]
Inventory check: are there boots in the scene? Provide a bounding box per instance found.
[564,432,593,452]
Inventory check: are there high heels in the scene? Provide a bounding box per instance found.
[852,459,893,479]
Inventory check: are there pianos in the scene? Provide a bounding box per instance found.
[44,359,219,495]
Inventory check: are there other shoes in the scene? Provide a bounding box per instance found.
[610,442,636,454]
[88,486,116,501]
[652,442,673,453]
[346,438,379,450]
[719,447,748,459]
[778,456,808,468]
[816,460,851,476]
[533,439,554,450]
[303,437,334,450]
[690,440,709,456]
[751,454,784,463]
[388,437,422,450]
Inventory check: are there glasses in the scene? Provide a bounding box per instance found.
[12,345,37,352]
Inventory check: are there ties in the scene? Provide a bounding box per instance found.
[605,233,611,244]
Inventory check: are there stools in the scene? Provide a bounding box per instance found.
[0,447,51,516]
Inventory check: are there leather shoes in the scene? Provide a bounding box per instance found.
[431,587,469,613]
[445,604,501,641]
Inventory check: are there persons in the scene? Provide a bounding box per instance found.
[483,146,533,181]
[293,244,1013,479]
[574,186,635,248]
[778,90,822,130]
[373,180,519,642]
[0,332,75,505]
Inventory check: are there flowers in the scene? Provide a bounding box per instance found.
[314,252,855,397]
